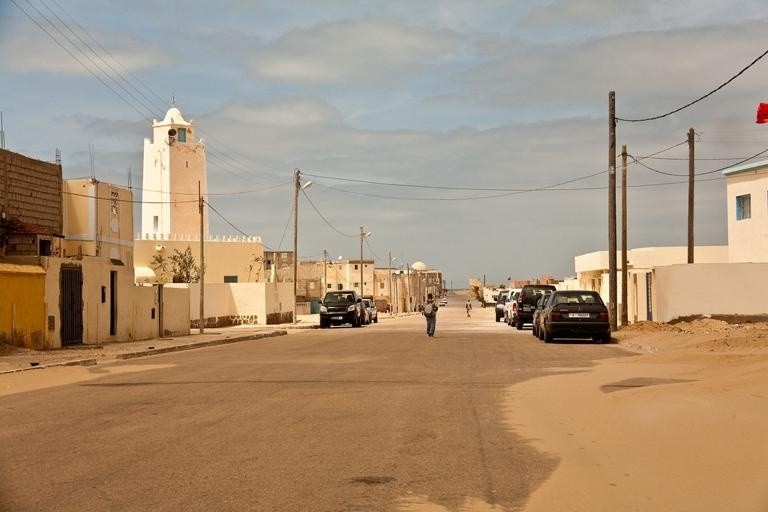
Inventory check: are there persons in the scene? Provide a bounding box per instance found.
[424,293,438,337]
[465,299,472,317]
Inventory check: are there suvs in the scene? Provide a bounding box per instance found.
[318,290,366,329]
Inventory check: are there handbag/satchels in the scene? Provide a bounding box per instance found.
[424,302,434,316]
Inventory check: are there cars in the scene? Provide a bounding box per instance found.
[439,298,447,306]
[362,299,378,324]
[493,284,610,344]
